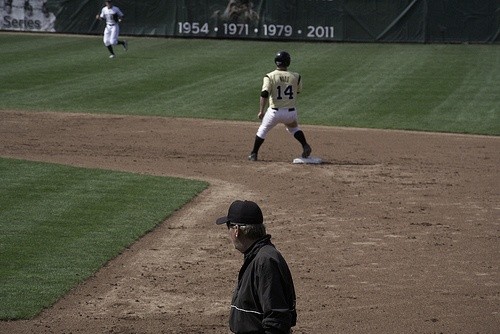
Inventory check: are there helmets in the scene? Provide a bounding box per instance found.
[274,51,291,67]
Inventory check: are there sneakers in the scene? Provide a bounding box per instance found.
[122,39,128,51]
[300,146,312,158]
[109,54,116,58]
[248,152,257,162]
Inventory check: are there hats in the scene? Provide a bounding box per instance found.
[216,200,264,226]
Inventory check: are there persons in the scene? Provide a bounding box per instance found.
[0,0,57,33]
[247,51,312,161]
[95,0,128,58]
[215,199,297,334]
